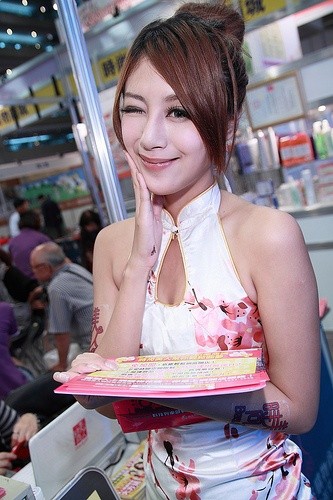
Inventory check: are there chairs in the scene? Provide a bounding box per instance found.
[7,285,52,378]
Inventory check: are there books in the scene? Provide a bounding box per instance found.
[53,345,270,399]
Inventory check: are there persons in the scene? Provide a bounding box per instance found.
[90,1,322,499]
[62,229,63,231]
[7,209,53,283]
[0,301,27,400]
[9,198,30,238]
[29,240,93,375]
[0,248,42,375]
[78,211,104,270]
[37,193,67,240]
[1,397,58,479]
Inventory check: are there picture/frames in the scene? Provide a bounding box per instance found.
[244,71,306,133]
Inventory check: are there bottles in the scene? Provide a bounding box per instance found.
[312,121,328,159]
[322,119,333,157]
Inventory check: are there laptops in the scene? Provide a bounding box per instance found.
[10,401,122,500]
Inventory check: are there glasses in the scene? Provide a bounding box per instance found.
[27,263,45,272]
